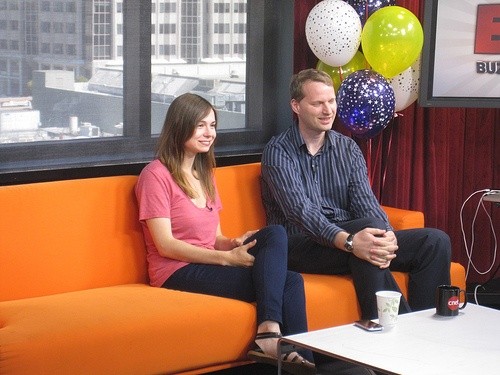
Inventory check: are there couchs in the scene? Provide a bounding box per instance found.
[0,163,466,375]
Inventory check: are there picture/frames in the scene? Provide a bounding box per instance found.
[417,0,500,109]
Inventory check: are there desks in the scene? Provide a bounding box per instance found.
[276,303,500,375]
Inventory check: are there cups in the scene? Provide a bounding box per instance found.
[435,285,467,316]
[375,290,402,327]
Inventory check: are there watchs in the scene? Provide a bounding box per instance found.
[344,235,354,253]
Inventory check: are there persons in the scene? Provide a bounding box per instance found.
[261,68,452,319]
[136,93,315,375]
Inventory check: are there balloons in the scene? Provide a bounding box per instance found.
[315,52,367,94]
[337,70,396,145]
[387,54,422,116]
[361,6,425,79]
[305,0,361,66]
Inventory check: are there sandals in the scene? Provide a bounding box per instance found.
[248,333,318,375]
